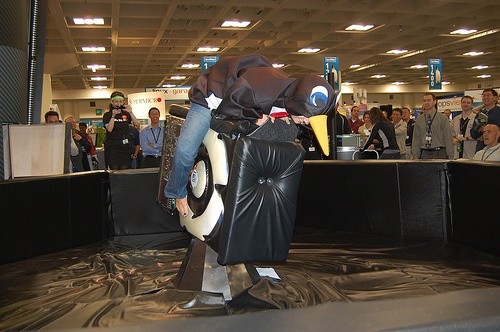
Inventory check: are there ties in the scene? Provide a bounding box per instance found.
[426,114,432,148]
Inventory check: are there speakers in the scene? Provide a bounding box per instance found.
[9,123,71,179]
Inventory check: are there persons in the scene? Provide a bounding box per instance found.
[382,110,388,119]
[413,93,455,161]
[165,54,337,219]
[396,106,416,160]
[470,89,500,154]
[474,124,500,162]
[347,105,363,134]
[389,107,407,160]
[103,92,139,171]
[419,104,436,115]
[46,110,100,173]
[140,106,165,169]
[449,96,478,160]
[443,108,453,122]
[359,111,375,134]
[334,101,351,144]
[360,107,401,160]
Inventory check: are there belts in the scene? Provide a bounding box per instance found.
[423,147,443,151]
[147,155,161,159]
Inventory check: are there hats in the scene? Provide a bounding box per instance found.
[64,115,74,122]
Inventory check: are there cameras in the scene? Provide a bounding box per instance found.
[117,105,126,112]
